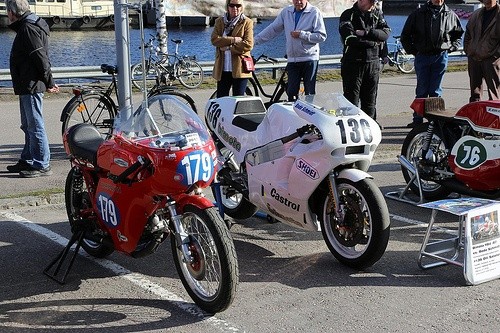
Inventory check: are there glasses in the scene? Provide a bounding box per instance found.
[228,3,242,8]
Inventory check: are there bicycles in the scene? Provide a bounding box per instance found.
[59,56,197,138]
[130,33,204,88]
[379,35,417,73]
[210,54,305,104]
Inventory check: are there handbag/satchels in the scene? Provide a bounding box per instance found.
[242,57,255,72]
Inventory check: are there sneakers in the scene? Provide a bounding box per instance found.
[20,165,52,177]
[7,159,31,171]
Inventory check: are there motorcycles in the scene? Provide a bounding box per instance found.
[43,93,240,315]
[204,93,391,269]
[387,96,500,203]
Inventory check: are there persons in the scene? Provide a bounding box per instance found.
[5,0,60,178]
[339,0,392,130]
[211,0,253,98]
[463,0,500,104]
[400,0,465,128]
[253,0,328,102]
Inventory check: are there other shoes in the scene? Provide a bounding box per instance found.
[407,118,423,128]
